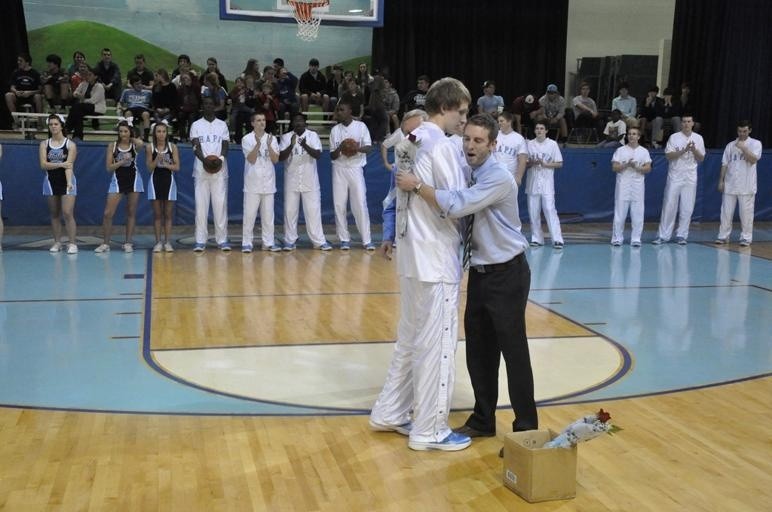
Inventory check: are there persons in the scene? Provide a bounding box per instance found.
[652,113,707,246]
[369,78,474,452]
[279,113,335,252]
[611,129,652,247]
[242,112,281,253]
[382,128,403,172]
[381,109,430,261]
[144,122,180,253]
[395,113,539,459]
[715,121,763,247]
[598,82,698,148]
[523,120,566,249]
[95,120,146,253]
[1,141,4,260]
[190,97,235,252]
[329,99,376,250]
[6,50,428,148]
[478,81,504,113]
[492,113,525,188]
[510,85,566,142]
[573,82,604,140]
[40,113,81,255]
[449,118,469,164]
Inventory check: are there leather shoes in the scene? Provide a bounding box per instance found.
[451,426,496,438]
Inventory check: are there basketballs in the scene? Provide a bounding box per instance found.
[205,155,220,173]
[342,138,357,158]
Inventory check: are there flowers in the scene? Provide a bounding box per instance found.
[593,406,615,426]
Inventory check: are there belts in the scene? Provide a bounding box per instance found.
[476,266,486,274]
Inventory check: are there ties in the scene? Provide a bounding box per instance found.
[461,172,476,267]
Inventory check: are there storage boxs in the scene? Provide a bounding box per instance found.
[502,429,578,505]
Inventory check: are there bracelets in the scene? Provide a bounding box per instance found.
[218,155,225,162]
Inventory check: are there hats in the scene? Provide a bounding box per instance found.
[547,84,558,92]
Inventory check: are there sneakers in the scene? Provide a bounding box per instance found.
[651,237,669,244]
[530,242,539,246]
[739,239,752,246]
[676,237,687,245]
[369,419,412,435]
[554,242,563,249]
[241,242,375,253]
[613,239,623,246]
[407,433,471,451]
[49,243,231,254]
[632,241,641,247]
[715,238,730,244]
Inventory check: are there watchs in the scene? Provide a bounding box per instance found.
[412,183,424,195]
